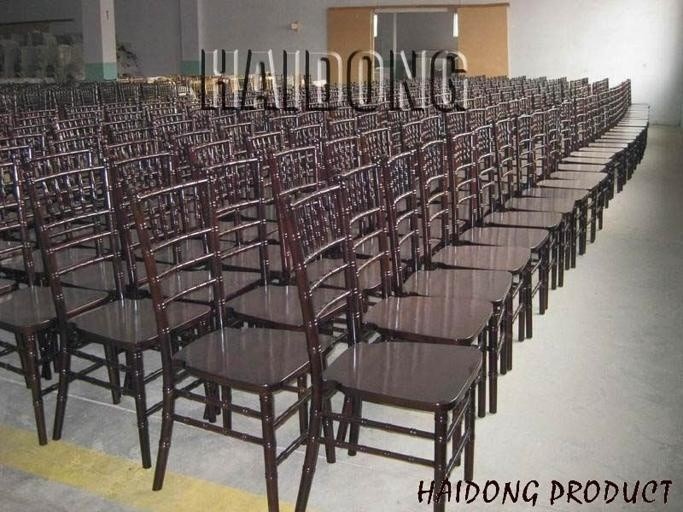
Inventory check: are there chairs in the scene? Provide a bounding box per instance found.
[1,76,649,511]
